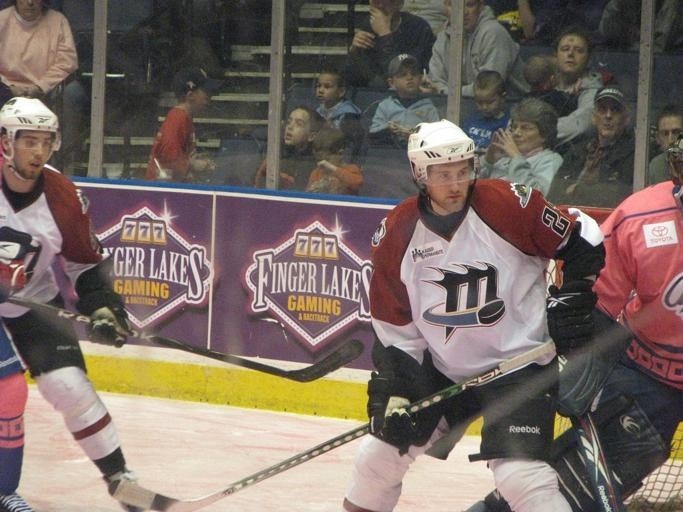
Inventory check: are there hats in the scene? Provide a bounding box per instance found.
[175,66,225,96]
[592,84,627,107]
[386,53,416,75]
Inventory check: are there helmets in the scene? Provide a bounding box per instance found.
[666,133,683,187]
[0,94,60,154]
[406,118,480,185]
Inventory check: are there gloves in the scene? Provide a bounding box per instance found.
[367,371,420,437]
[79,291,136,348]
[548,287,597,343]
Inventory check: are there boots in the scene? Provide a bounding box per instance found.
[104,469,146,512]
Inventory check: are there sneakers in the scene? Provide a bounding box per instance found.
[0,489,34,511]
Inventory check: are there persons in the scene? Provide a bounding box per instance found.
[0,0,683,211]
[464,134,683,512]
[0,95,150,511]
[339,120,605,512]
[0,323,30,512]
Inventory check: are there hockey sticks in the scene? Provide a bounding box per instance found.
[108,325,558,512]
[6,294,364,382]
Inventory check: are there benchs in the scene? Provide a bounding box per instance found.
[287,84,518,150]
[512,46,682,106]
[214,138,627,209]
